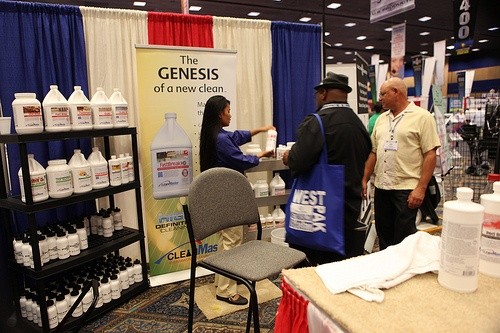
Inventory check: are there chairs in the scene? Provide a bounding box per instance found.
[182,167,309,333]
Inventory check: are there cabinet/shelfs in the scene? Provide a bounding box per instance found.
[0,126,149,333]
[241,157,292,242]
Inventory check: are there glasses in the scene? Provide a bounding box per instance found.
[312,88,324,96]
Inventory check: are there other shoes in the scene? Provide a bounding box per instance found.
[216,294,248,305]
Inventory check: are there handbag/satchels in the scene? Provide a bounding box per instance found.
[283,163,346,256]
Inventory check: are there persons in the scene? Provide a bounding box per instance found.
[361,77,441,251]
[282,72,372,269]
[199,95,276,306]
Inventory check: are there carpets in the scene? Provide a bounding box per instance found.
[194,278,282,320]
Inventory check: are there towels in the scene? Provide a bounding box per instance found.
[312,230,442,304]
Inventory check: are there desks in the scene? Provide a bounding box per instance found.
[274,230,500,333]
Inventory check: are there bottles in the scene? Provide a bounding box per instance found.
[87,147,109,190]
[285,141,296,154]
[18,154,49,204]
[108,88,129,128]
[12,93,43,134]
[150,112,193,200]
[246,144,262,162]
[46,159,73,199]
[437,187,484,294]
[109,153,134,187]
[68,149,92,194]
[43,85,71,132]
[90,87,113,130]
[248,206,286,233]
[68,86,93,132]
[270,173,285,196]
[477,174,500,279]
[254,180,269,199]
[12,206,143,329]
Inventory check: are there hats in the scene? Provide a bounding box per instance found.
[313,71,352,93]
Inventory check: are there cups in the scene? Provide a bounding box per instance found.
[0,117,11,135]
